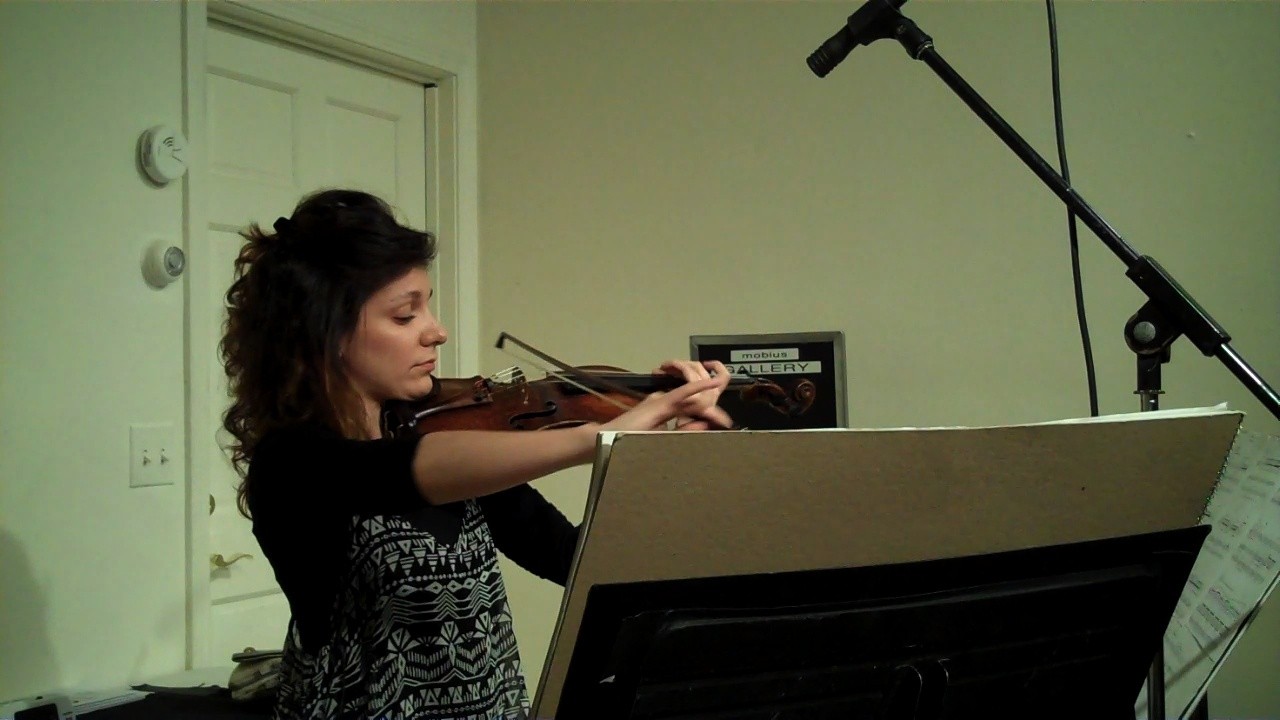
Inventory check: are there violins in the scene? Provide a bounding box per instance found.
[399,362,817,439]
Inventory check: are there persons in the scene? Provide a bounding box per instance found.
[214,188,732,720]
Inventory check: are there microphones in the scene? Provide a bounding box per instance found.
[805,0,908,79]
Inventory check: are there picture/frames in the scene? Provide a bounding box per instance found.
[689,329,847,431]
